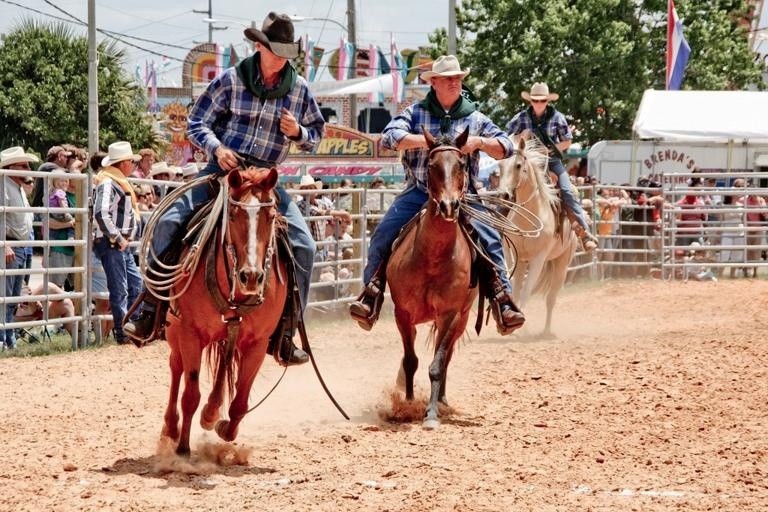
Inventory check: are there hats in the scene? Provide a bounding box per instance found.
[244,11,303,59]
[0,146,39,168]
[48,146,72,158]
[419,54,466,82]
[521,82,559,101]
[101,141,142,168]
[151,161,199,178]
[293,175,323,196]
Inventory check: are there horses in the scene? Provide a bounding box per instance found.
[158,167,289,461]
[385,124,478,430]
[497,128,578,337]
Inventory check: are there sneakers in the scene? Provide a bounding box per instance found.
[350,300,375,331]
[500,309,525,335]
[125,311,155,336]
[585,241,596,250]
[266,335,309,363]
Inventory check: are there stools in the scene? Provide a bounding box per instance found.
[12,317,52,346]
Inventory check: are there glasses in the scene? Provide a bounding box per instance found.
[532,99,547,103]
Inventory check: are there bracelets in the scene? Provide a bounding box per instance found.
[478,135,485,149]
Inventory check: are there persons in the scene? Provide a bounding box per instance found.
[123,12,326,363]
[564,166,768,283]
[478,168,500,206]
[0,142,198,352]
[295,175,408,322]
[503,82,598,253]
[350,55,525,336]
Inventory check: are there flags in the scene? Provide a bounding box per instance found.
[666,0,692,90]
[215,33,409,104]
[134,56,171,114]
